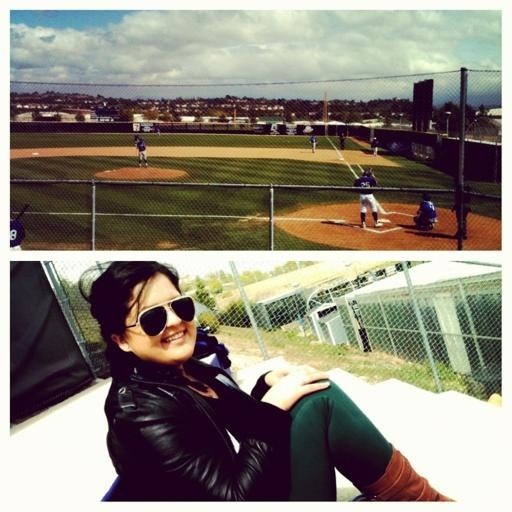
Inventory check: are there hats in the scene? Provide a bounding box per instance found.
[363,170,371,176]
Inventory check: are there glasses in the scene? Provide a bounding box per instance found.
[124,295,195,337]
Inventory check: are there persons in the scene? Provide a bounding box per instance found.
[134,134,140,148]
[390,141,399,157]
[353,171,384,228]
[450,185,471,240]
[309,134,318,153]
[154,125,161,137]
[371,137,379,157]
[339,133,346,150]
[137,138,148,167]
[77,259,458,500]
[11,219,27,250]
[413,193,437,231]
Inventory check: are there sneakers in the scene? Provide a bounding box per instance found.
[374,222,383,227]
[361,222,367,228]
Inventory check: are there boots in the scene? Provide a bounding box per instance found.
[359,441,455,502]
